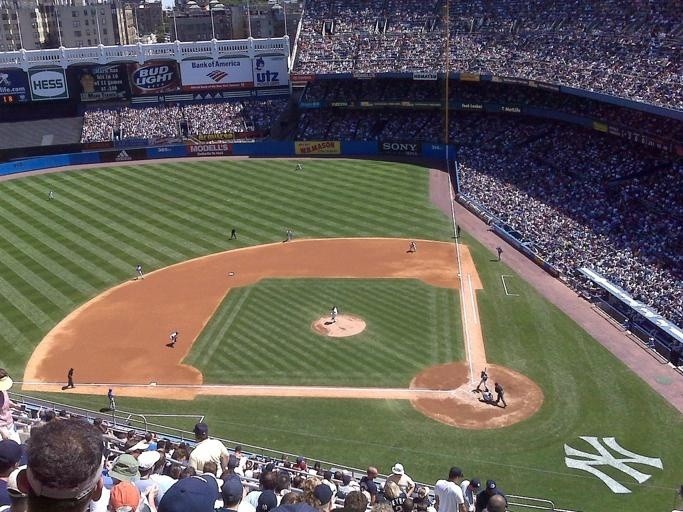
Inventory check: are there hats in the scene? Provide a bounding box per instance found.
[0,440,27,465]
[256,492,277,512]
[108,454,141,483]
[0,374,14,391]
[471,479,481,490]
[221,474,243,502]
[226,446,308,475]
[486,480,496,493]
[193,423,208,435]
[137,450,160,471]
[313,484,333,506]
[6,464,28,498]
[450,467,464,477]
[391,463,404,475]
[109,480,140,512]
[157,474,219,512]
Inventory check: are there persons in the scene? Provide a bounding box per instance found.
[80,95,290,143]
[1,368,509,511]
[169,330,178,347]
[408,242,415,252]
[282,228,296,243]
[134,264,144,280]
[291,0,683,145]
[454,142,683,330]
[228,227,237,240]
[329,306,336,323]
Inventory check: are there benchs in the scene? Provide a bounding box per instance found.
[77,94,287,145]
